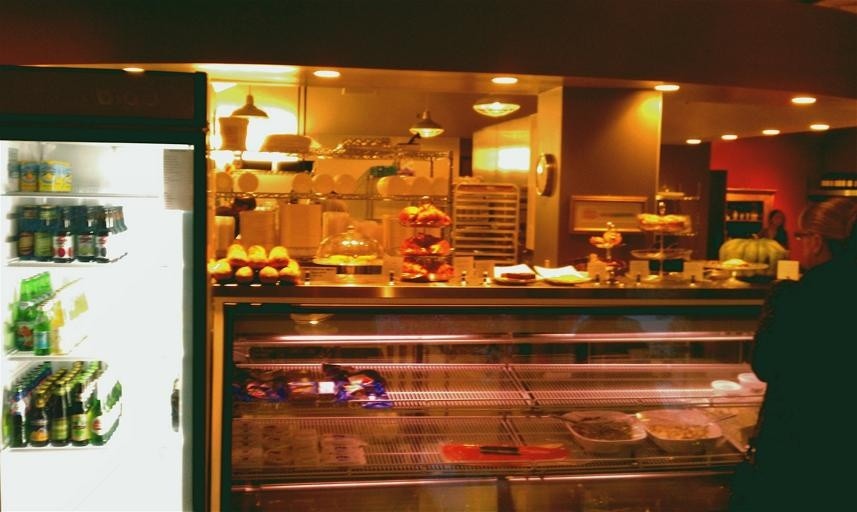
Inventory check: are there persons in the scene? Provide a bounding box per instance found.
[727,196,857,511]
[752,208,788,249]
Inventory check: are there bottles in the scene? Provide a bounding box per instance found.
[5,273,93,357]
[6,360,125,448]
[16,200,131,262]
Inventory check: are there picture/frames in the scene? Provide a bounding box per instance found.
[568,192,652,238]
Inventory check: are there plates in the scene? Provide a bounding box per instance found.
[288,313,332,326]
[264,133,312,154]
[493,263,593,286]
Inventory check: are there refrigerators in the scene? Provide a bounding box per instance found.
[1,60,213,511]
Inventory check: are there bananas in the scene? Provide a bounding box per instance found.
[718,239,788,282]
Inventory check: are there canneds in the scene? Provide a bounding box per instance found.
[19,162,75,193]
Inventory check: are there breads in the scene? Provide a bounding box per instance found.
[230,420,368,468]
[210,243,300,285]
[396,206,455,283]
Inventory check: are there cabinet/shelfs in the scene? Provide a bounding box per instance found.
[204,276,769,512]
[453,182,529,268]
[207,151,454,264]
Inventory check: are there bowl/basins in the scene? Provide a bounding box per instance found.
[560,406,725,455]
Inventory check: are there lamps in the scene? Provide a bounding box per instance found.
[230,84,268,121]
[471,94,523,121]
[406,91,447,141]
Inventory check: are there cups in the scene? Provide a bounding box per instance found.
[218,115,249,151]
[710,370,766,399]
